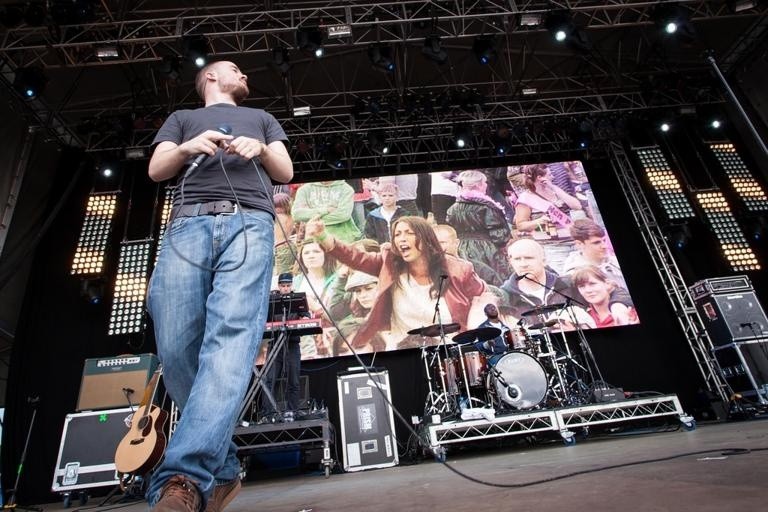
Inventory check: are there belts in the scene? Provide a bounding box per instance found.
[169,199,238,216]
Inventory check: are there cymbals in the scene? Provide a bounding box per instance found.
[407,323,460,337]
[521,302,573,316]
[452,328,501,343]
[529,322,556,329]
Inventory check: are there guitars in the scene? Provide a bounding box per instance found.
[114,364,170,474]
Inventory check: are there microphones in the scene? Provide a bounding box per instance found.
[441,274,449,279]
[122,387,135,395]
[192,123,233,167]
[508,385,518,399]
[516,272,530,282]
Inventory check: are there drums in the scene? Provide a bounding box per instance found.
[486,351,548,410]
[442,357,462,396]
[533,336,548,353]
[505,328,531,351]
[462,352,486,387]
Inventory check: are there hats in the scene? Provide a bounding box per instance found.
[279,273,292,283]
[344,270,379,292]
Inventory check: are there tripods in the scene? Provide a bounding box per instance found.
[525,277,630,406]
[418,334,443,416]
[449,340,487,409]
[429,336,456,414]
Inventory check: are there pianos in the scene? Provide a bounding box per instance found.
[264,319,323,339]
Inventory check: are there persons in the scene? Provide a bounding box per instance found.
[258,273,311,425]
[143,60,293,512]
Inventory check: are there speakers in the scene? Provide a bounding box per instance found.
[74,352,157,414]
[50,405,142,493]
[694,291,768,344]
[713,338,768,407]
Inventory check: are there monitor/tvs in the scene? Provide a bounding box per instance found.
[268,291,308,318]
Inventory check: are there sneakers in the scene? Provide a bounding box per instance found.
[202,476,242,511]
[149,474,204,511]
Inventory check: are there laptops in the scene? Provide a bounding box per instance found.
[347,350,377,372]
